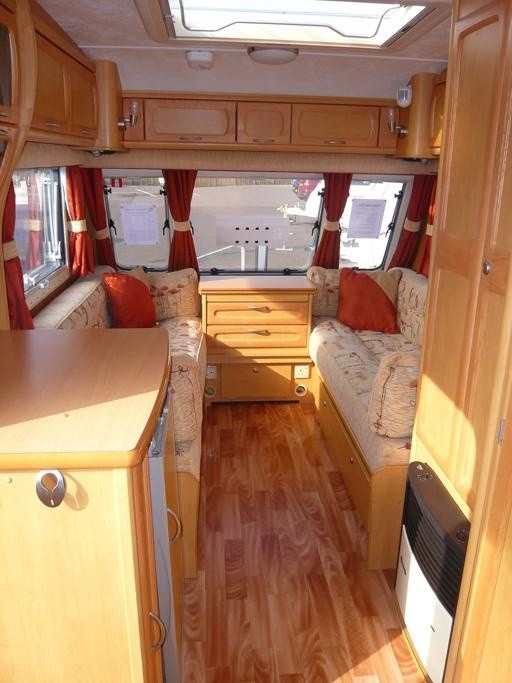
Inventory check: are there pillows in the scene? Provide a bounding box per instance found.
[96,265,156,328]
[333,265,401,338]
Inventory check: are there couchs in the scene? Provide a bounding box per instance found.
[301,265,432,576]
[23,259,212,584]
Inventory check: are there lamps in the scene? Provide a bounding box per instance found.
[384,108,409,140]
[245,41,301,64]
[115,94,139,132]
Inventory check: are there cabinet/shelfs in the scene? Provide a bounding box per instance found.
[131,92,237,144]
[408,25,512,683]
[70,43,104,146]
[234,96,293,148]
[198,273,319,408]
[420,77,446,154]
[0,27,40,329]
[0,325,180,681]
[295,90,406,156]
[20,34,72,147]
